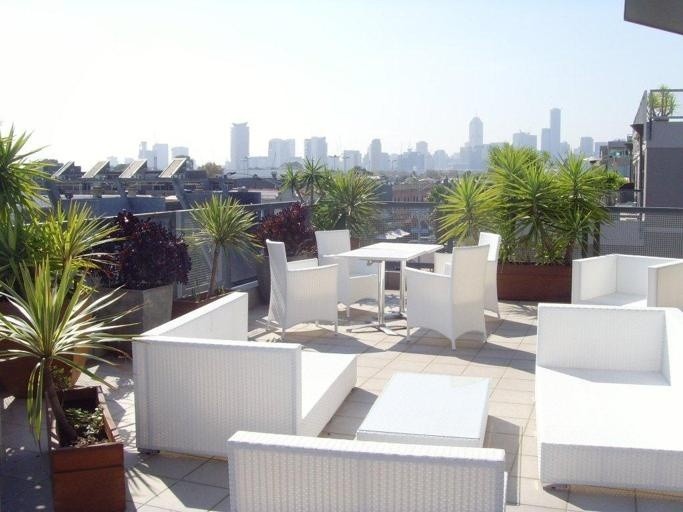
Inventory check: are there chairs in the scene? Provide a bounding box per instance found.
[264,239,339,339]
[402,244,492,351]
[433,230,503,319]
[570,252,682,311]
[226,431,511,512]
[314,229,382,322]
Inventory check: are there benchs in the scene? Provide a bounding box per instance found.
[129,291,357,459]
[536,305,621,491]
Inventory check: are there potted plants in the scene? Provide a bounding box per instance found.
[276,155,387,249]
[174,193,265,318]
[2,253,154,511]
[84,208,189,357]
[429,142,633,303]
[647,85,676,122]
[1,121,62,396]
[254,201,319,302]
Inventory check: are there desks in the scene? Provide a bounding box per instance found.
[352,370,492,448]
[325,242,445,334]
